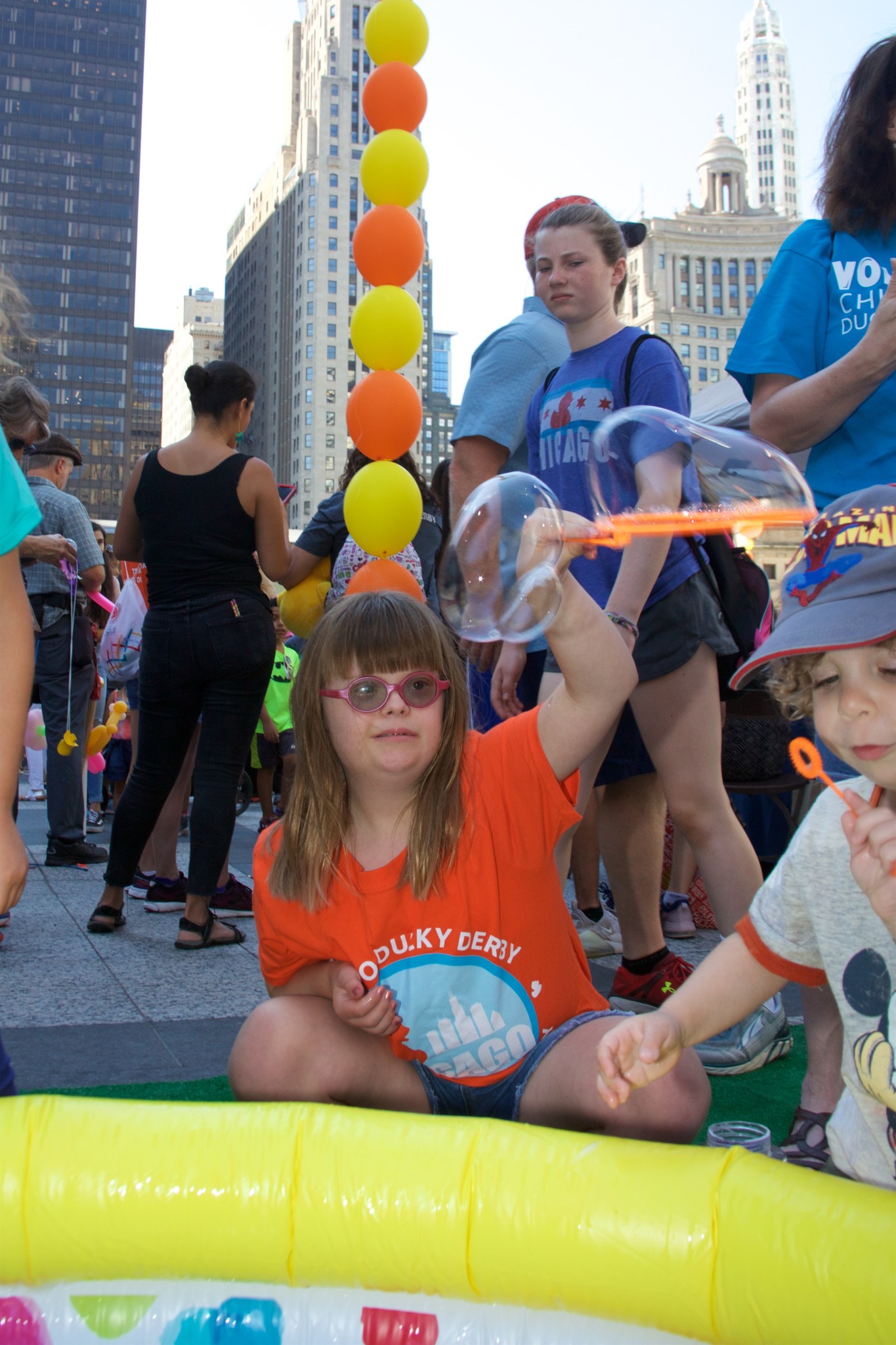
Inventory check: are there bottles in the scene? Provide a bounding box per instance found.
[707,1121,788,1164]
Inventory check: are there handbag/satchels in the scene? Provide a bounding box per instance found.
[101,560,147,682]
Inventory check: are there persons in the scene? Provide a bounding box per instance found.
[487,201,792,1073]
[443,192,695,1008]
[12,361,810,960]
[722,32,896,1163]
[228,506,713,1140]
[593,483,896,1189]
[0,270,43,1097]
[0,369,80,579]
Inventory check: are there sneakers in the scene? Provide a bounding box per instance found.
[570,898,623,958]
[143,871,187,913]
[661,901,696,937]
[43,838,109,866]
[124,867,156,899]
[207,873,254,918]
[607,950,694,1014]
[86,809,105,833]
[691,992,794,1076]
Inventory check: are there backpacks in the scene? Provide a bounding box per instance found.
[695,524,774,695]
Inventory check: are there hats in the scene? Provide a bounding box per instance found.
[729,484,896,688]
[524,195,646,261]
[30,430,84,466]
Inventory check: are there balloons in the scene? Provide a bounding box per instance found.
[344,558,424,603]
[366,1,428,64]
[344,460,424,558]
[346,369,422,460]
[362,60,428,133]
[353,204,425,287]
[360,126,427,208]
[347,285,423,370]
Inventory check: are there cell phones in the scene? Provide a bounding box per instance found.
[276,484,298,506]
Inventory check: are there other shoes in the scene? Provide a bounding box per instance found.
[258,813,279,833]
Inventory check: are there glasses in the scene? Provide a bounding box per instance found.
[320,671,449,713]
[8,437,36,455]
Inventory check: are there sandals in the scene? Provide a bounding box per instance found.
[87,899,127,932]
[175,907,246,950]
[18,790,46,801]
[780,1104,835,1171]
